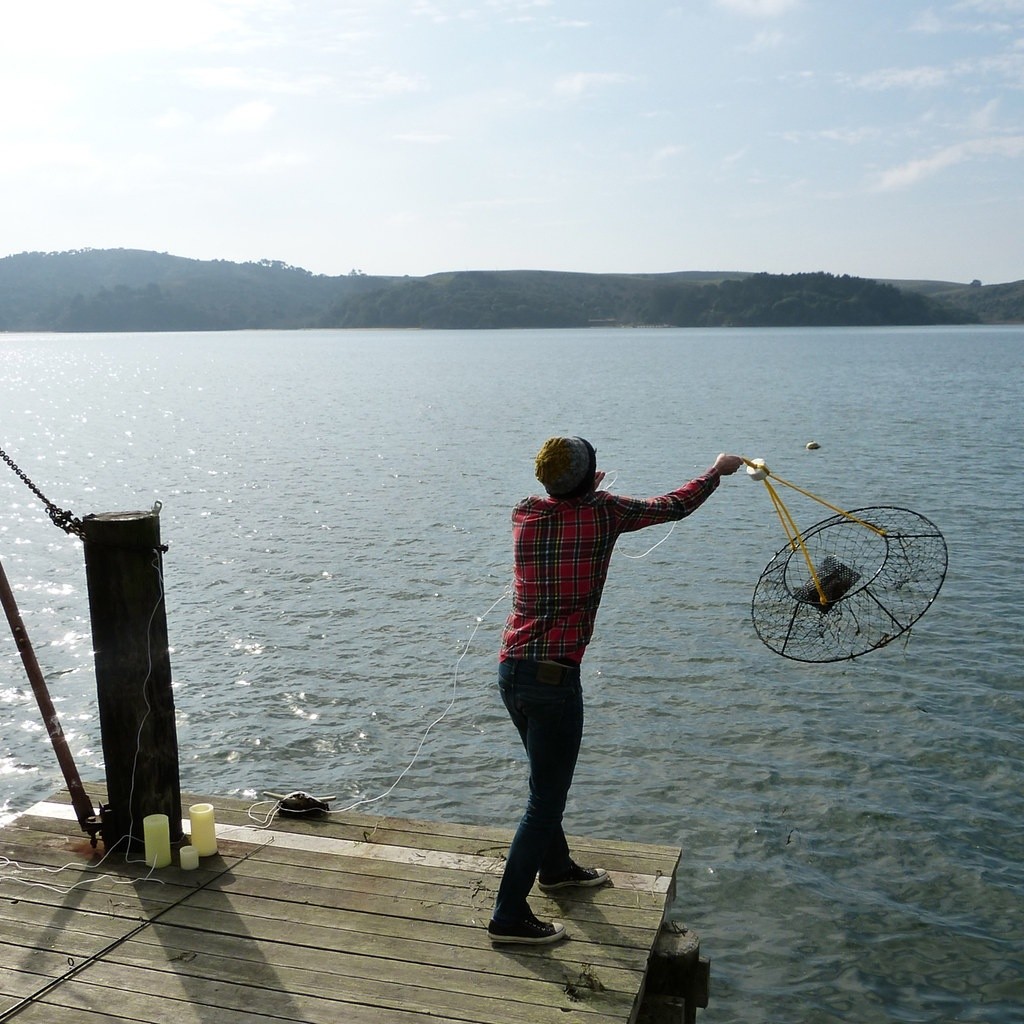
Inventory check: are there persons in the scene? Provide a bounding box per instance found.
[484,432,745,947]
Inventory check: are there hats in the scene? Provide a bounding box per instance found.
[534,437,589,498]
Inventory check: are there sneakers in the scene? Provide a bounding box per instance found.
[487,910,566,945]
[537,856,608,890]
